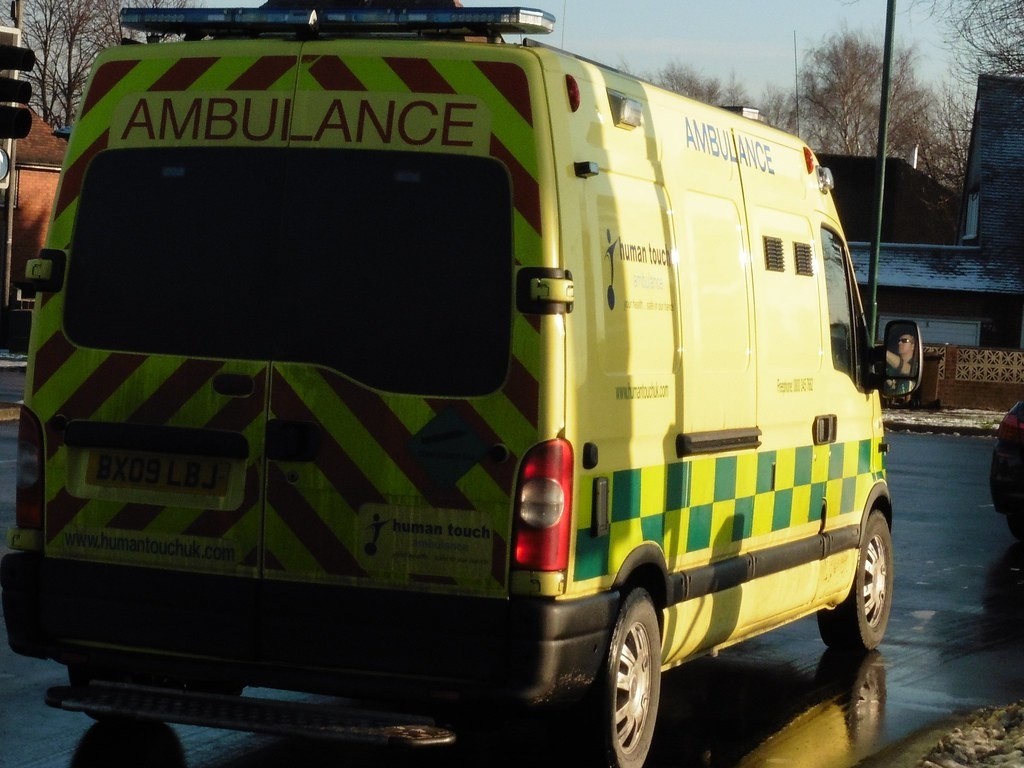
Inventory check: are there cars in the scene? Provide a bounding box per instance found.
[990,398,1024,540]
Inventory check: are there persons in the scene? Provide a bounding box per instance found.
[884,379,906,396]
[886,333,916,375]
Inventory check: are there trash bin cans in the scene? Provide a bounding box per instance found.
[917,352,944,408]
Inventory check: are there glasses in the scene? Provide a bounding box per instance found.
[896,338,910,344]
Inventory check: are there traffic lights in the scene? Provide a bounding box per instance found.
[0,42,36,141]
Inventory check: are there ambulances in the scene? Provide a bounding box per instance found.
[0,0,926,768]
